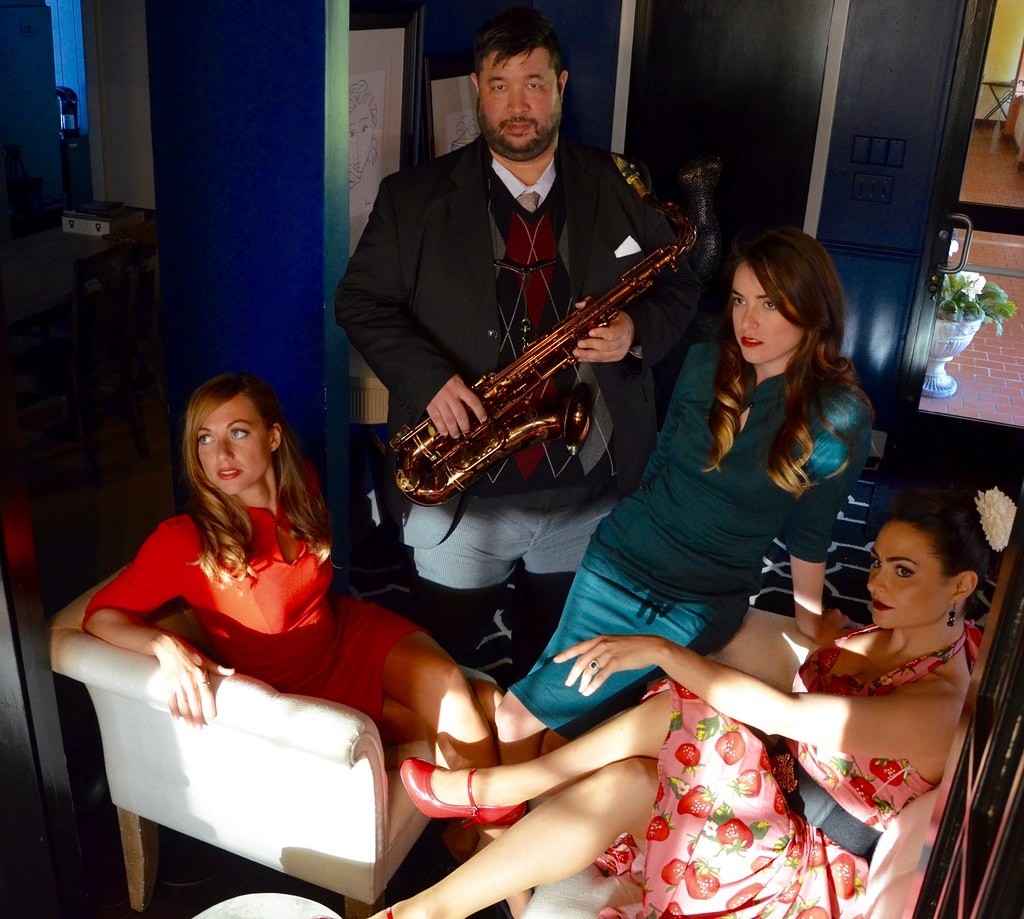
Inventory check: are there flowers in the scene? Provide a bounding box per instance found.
[975,484,1014,551]
[935,271,1017,337]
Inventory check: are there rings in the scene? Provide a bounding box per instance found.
[589,661,600,670]
[197,682,210,687]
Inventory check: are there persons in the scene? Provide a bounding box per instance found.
[335,11,700,681]
[373,488,1017,919]
[494,229,871,812]
[82,373,533,919]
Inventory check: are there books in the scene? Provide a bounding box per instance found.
[74,201,126,216]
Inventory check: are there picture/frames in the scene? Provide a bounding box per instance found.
[349,0,425,261]
[424,53,481,159]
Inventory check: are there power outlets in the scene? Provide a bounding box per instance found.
[851,173,894,204]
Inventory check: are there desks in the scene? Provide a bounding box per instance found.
[976,82,1018,129]
[0,225,154,325]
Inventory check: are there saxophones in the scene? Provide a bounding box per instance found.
[387,150,700,507]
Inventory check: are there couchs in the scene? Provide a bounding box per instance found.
[518,608,944,919]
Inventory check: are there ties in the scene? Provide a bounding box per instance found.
[516,191,540,213]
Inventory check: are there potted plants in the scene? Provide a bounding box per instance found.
[922,318,984,399]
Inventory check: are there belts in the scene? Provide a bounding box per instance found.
[768,735,883,857]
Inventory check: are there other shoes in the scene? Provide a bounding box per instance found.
[437,822,481,865]
[501,887,534,919]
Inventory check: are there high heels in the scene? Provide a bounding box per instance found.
[400,756,526,828]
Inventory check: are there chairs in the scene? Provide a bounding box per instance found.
[6,242,157,489]
[47,562,497,919]
